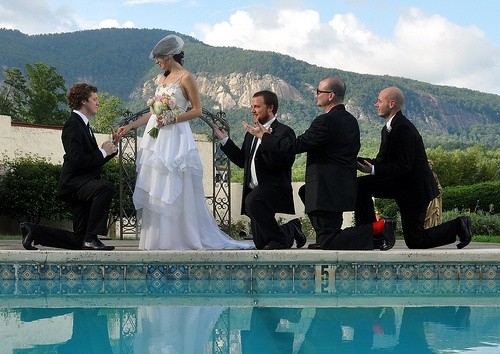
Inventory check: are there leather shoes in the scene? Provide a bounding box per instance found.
[20,222,38,250]
[82,239,114,250]
[455,216,471,248]
[264,241,286,250]
[288,218,306,248]
[308,243,320,249]
[374,240,383,248]
[380,219,396,250]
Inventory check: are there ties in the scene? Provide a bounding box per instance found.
[247,137,258,182]
[386,130,388,137]
[87,122,89,135]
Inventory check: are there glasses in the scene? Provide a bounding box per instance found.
[317,89,336,95]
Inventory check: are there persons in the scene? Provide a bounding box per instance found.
[242,78,397,251]
[395,306,471,354]
[13,308,113,354]
[117,37,256,251]
[354,87,471,249]
[20,83,122,250]
[132,307,229,354]
[213,91,306,250]
[240,307,303,354]
[298,307,396,354]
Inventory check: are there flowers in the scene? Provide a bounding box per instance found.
[147,93,181,139]
